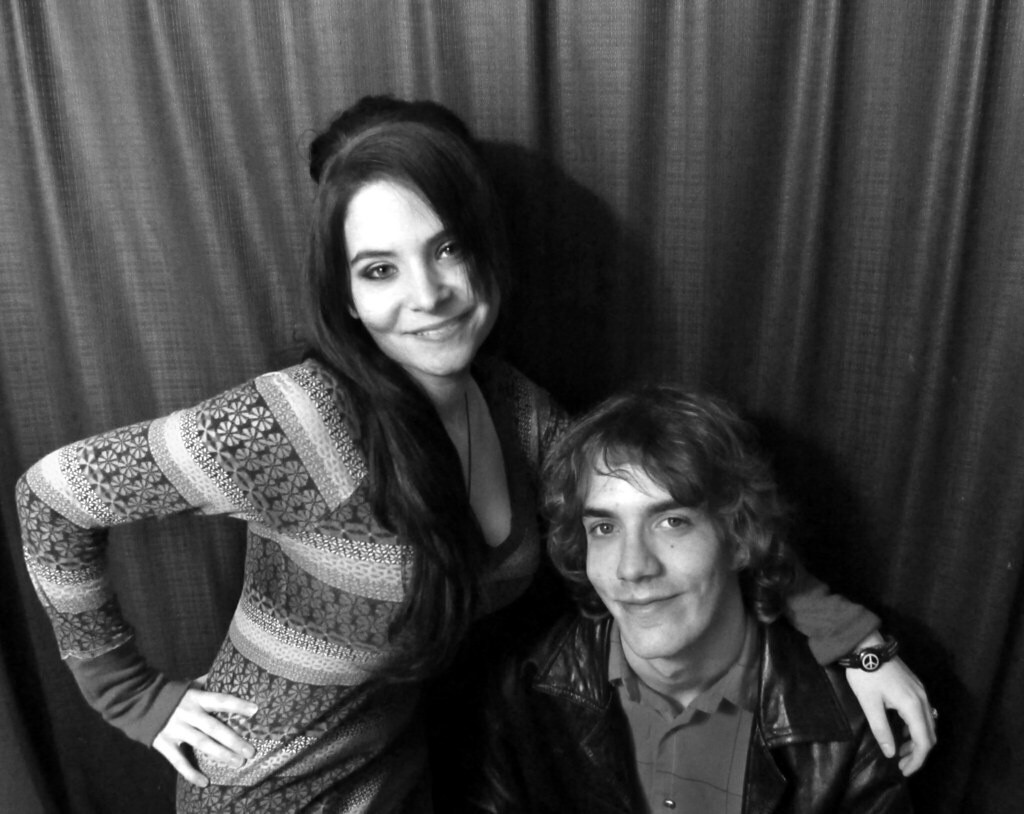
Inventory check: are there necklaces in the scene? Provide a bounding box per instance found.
[464,390,471,502]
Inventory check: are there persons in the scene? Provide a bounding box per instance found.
[16,101,939,814]
[434,385,937,814]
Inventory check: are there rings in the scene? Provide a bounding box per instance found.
[931,708,937,718]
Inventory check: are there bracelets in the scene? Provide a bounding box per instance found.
[837,635,897,672]
